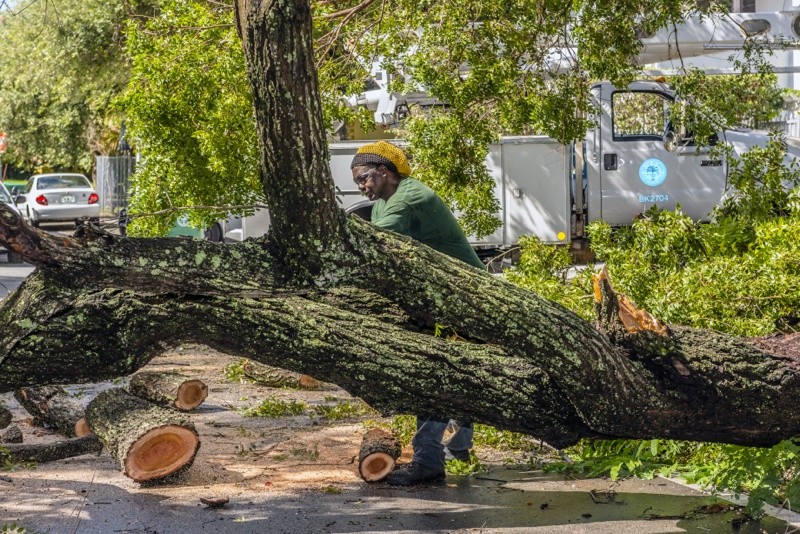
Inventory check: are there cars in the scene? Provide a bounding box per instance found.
[11,173,100,228]
[0,182,22,264]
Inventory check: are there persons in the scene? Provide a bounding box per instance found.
[351,141,486,485]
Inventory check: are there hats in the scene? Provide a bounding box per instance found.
[351,141,411,177]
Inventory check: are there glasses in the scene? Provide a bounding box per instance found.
[353,164,381,185]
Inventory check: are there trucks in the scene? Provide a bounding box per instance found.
[221,79,800,254]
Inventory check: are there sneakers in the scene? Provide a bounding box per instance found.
[442,445,470,462]
[386,463,445,485]
